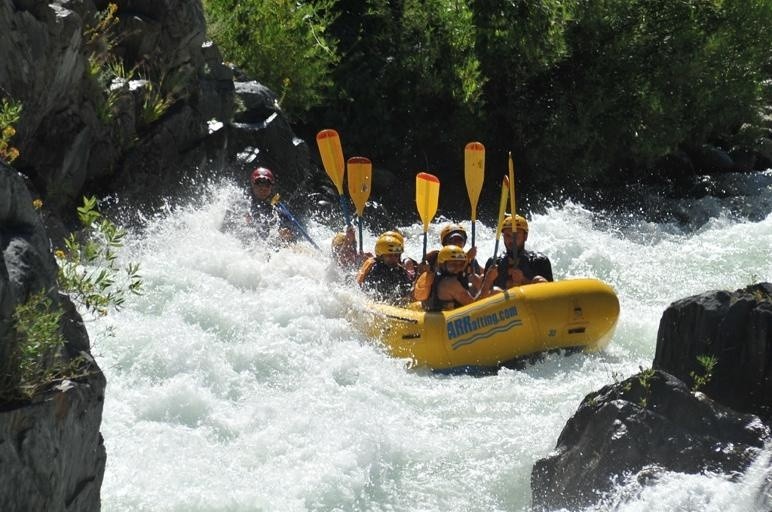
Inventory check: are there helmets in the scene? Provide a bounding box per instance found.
[440,225,468,248]
[437,245,467,265]
[501,216,529,233]
[250,167,275,186]
[379,231,404,245]
[374,237,404,255]
[333,233,349,247]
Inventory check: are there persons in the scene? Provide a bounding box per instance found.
[418,225,486,295]
[484,216,552,298]
[358,236,430,308]
[325,225,373,289]
[220,168,300,244]
[415,245,497,313]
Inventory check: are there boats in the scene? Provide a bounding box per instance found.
[279,242,620,378]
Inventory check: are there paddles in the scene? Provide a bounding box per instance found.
[493,175,510,264]
[440,237,441,238]
[508,151,518,268]
[317,130,357,260]
[348,157,371,254]
[465,142,485,273]
[416,172,440,264]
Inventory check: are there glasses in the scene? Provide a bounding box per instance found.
[253,178,271,188]
